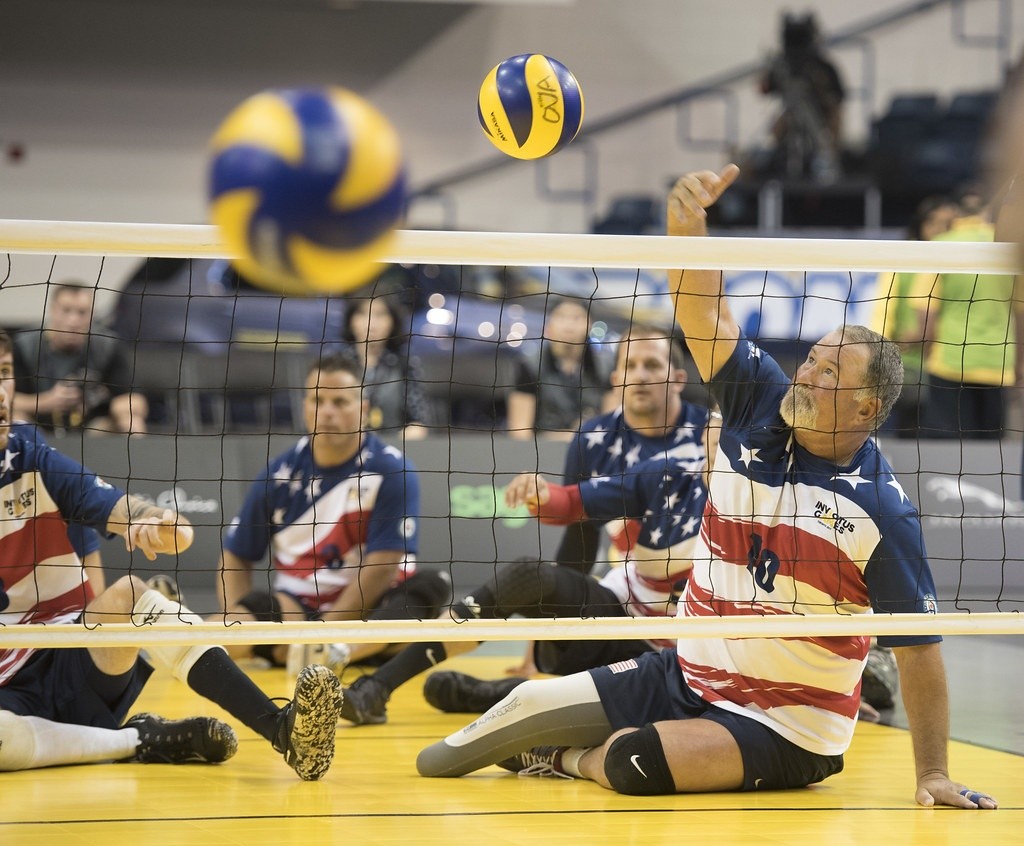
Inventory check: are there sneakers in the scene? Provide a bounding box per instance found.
[498,742,575,780]
[254,662,344,780]
[340,674,390,723]
[860,644,897,703]
[422,672,484,714]
[113,711,237,764]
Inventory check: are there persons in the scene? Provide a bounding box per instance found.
[207,354,456,678]
[1,324,344,783]
[873,194,1018,440]
[13,280,151,440]
[321,280,456,439]
[416,165,998,814]
[338,323,714,726]
[506,293,620,442]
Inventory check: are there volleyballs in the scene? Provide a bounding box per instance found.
[474,51,587,163]
[201,82,411,294]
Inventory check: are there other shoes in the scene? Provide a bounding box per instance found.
[286,640,330,676]
